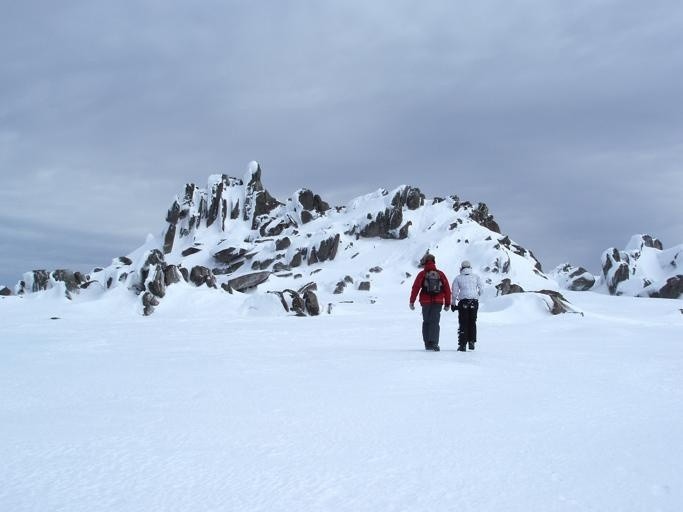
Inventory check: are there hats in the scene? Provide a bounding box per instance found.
[461,260,470,268]
[423,255,436,264]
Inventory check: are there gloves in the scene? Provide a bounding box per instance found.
[444,304,450,311]
[451,305,457,311]
[409,303,415,310]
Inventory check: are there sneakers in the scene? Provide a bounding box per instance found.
[457,340,474,352]
[426,344,441,352]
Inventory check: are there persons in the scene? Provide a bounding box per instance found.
[409,254,451,352]
[451,260,484,352]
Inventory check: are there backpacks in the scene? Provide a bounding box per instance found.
[420,269,443,294]
